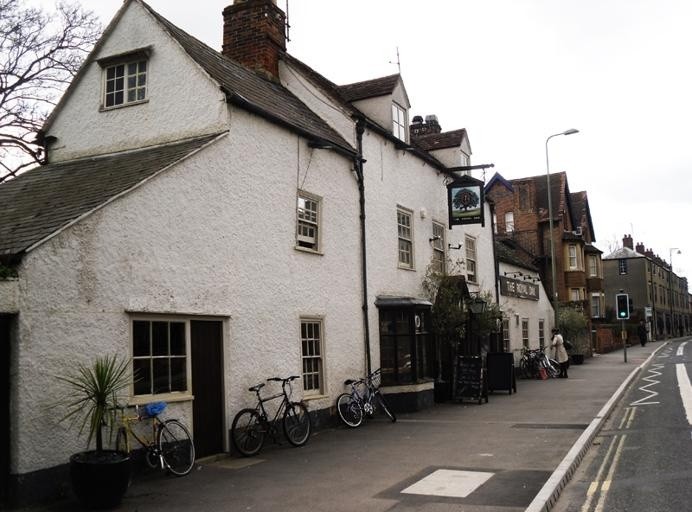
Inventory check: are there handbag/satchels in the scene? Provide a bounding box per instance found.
[563,340,573,349]
[540,367,548,380]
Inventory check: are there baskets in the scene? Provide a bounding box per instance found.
[365,372,385,391]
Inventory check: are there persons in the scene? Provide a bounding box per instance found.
[638,319,649,347]
[550,327,569,378]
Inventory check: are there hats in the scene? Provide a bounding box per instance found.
[552,328,559,335]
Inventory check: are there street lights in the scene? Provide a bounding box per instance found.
[669,247,681,337]
[546,128,579,332]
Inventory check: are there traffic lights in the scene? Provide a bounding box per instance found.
[616,294,629,320]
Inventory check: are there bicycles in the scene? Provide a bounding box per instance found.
[337,368,395,428]
[515,346,561,380]
[232,376,311,456]
[109,402,196,477]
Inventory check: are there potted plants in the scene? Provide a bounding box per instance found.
[41,351,146,510]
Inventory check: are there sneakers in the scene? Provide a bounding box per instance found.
[556,374,568,378]
